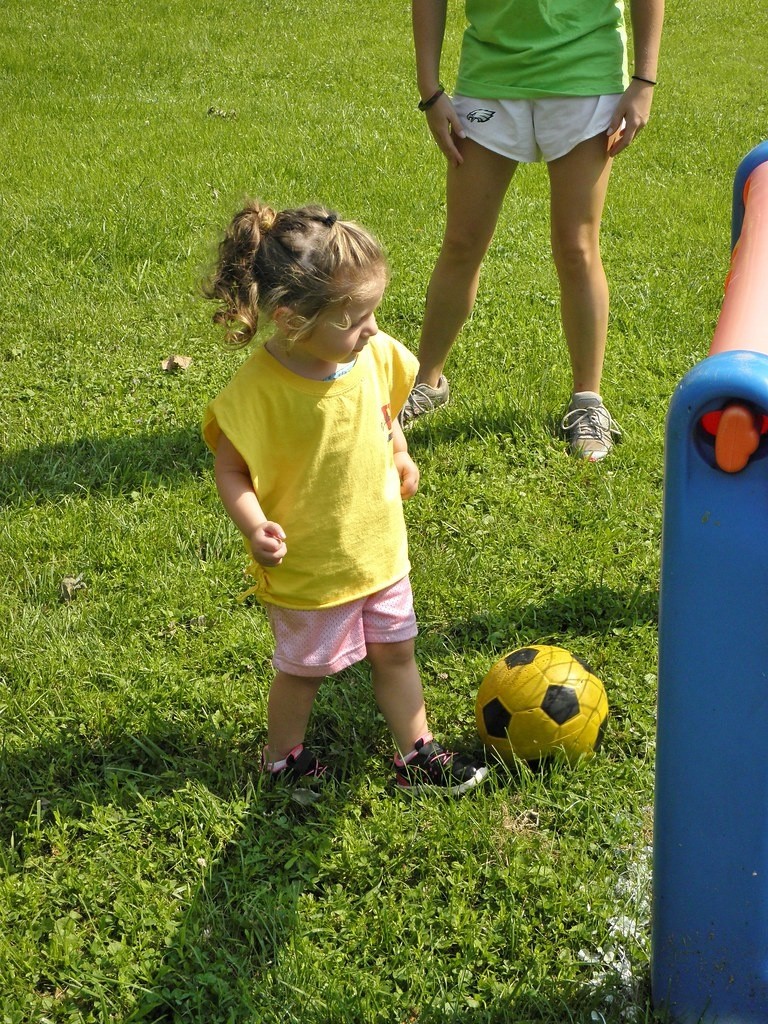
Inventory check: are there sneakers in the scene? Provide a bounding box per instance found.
[561,391,622,463]
[398,374,449,428]
[261,744,357,815]
[392,739,489,797]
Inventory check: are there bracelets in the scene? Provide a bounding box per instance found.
[418,85,443,110]
[632,76,657,85]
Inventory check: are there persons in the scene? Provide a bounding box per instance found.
[202,205,489,794]
[398,0,666,460]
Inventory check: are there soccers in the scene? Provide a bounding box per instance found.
[476,644,609,774]
